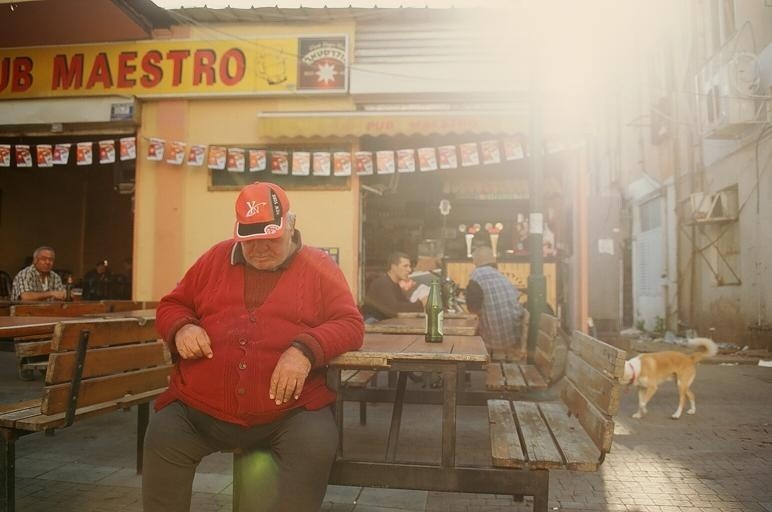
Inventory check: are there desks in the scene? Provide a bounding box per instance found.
[324,311,548,512]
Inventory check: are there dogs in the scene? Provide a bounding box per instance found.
[617,337,718,419]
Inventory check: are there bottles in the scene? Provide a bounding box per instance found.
[66,271,73,302]
[424,278,446,343]
[447,285,454,311]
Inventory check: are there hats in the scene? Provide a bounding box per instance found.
[473,247,496,266]
[234,181,289,240]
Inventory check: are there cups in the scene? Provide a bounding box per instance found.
[71,287,83,303]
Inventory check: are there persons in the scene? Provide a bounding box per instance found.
[141,181,365,512]
[464,246,525,352]
[358,252,429,325]
[10,246,133,382]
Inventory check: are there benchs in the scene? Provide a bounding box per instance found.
[1,301,173,511]
[486,308,628,510]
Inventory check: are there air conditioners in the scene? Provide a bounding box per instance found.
[700,60,755,137]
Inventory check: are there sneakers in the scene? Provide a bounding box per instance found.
[18,369,35,380]
